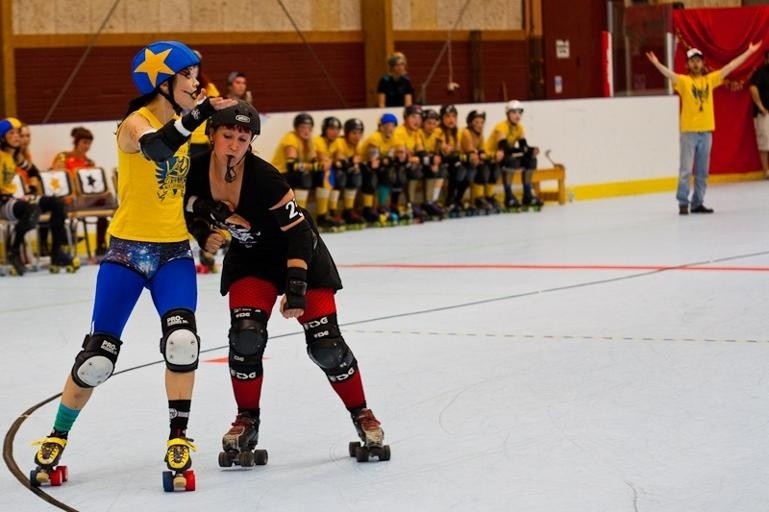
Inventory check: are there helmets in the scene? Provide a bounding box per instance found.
[345,118,364,133]
[381,113,398,125]
[440,103,458,114]
[505,99,524,113]
[1,117,22,135]
[422,109,439,119]
[293,113,313,127]
[404,105,422,119]
[131,40,200,96]
[466,110,487,120]
[204,99,262,136]
[322,117,342,130]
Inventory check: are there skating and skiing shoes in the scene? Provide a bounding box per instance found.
[49,248,81,275]
[161,438,196,492]
[349,407,390,462]
[219,408,268,467]
[28,428,68,486]
[317,191,544,233]
[195,249,219,273]
[6,245,25,277]
[92,247,109,263]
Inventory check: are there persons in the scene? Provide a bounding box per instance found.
[222,71,252,104]
[646,40,762,214]
[190,50,221,145]
[183,97,384,452]
[34,40,238,472]
[749,49,769,179]
[377,52,414,107]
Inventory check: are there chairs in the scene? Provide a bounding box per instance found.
[1,165,123,274]
[503,148,568,207]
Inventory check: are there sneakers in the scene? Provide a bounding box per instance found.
[678,203,689,214]
[691,204,714,213]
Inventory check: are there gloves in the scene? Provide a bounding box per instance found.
[310,146,541,174]
[193,95,218,118]
[196,228,220,252]
[283,268,307,311]
[212,201,234,225]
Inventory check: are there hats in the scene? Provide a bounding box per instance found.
[687,48,703,57]
[71,127,93,143]
[388,52,404,60]
[227,71,246,82]
